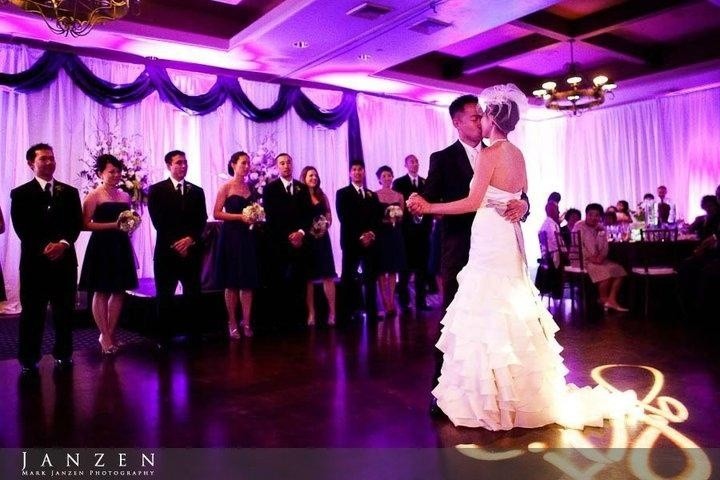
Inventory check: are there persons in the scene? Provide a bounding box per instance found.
[1,83,719,432]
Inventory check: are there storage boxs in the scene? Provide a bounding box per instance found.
[608,220,701,261]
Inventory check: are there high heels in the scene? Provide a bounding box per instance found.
[599,298,630,312]
[99,334,119,355]
[229,321,241,339]
[240,321,253,337]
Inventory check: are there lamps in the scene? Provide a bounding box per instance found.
[9,0,130,39]
[532,36,618,117]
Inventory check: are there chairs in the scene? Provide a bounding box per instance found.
[633,227,679,316]
[537,231,553,308]
[552,231,595,310]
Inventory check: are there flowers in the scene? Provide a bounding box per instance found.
[116,209,141,235]
[630,201,653,221]
[385,204,404,226]
[242,201,269,228]
[75,104,153,217]
[215,124,280,195]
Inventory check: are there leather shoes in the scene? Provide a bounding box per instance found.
[22,365,35,375]
[57,359,72,368]
[366,314,384,320]
[417,304,432,310]
[155,341,166,353]
[430,397,446,421]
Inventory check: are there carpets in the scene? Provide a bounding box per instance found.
[1,314,146,358]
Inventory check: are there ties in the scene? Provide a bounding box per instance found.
[176,184,182,212]
[359,189,363,199]
[469,149,478,171]
[413,179,416,186]
[45,183,51,207]
[286,183,291,198]
